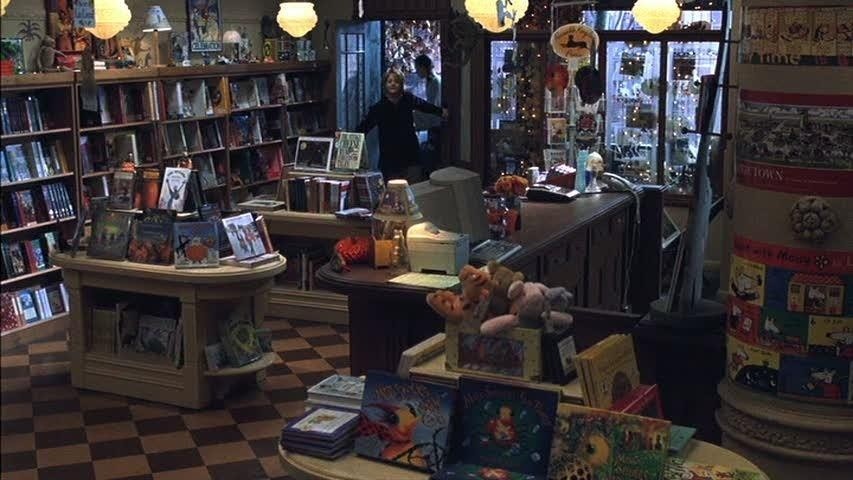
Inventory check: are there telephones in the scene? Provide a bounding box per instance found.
[600,172,634,192]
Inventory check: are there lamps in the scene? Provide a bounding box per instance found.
[141,7,172,64]
[464,1,529,33]
[373,179,423,281]
[276,1,319,38]
[631,1,683,35]
[83,0,132,40]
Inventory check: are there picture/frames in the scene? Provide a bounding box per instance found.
[187,0,223,53]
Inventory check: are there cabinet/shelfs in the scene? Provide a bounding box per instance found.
[0,74,77,353]
[280,59,330,167]
[52,254,286,412]
[505,200,636,313]
[165,64,231,213]
[228,63,283,204]
[248,208,373,326]
[76,69,165,236]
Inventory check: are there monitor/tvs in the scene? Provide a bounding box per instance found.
[406,166,490,241]
[295,135,333,171]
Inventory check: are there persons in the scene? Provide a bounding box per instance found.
[56,2,88,52]
[413,54,444,153]
[334,67,448,181]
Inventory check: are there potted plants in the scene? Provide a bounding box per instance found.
[0,38,21,76]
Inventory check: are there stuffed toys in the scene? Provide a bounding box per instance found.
[426,259,572,333]
